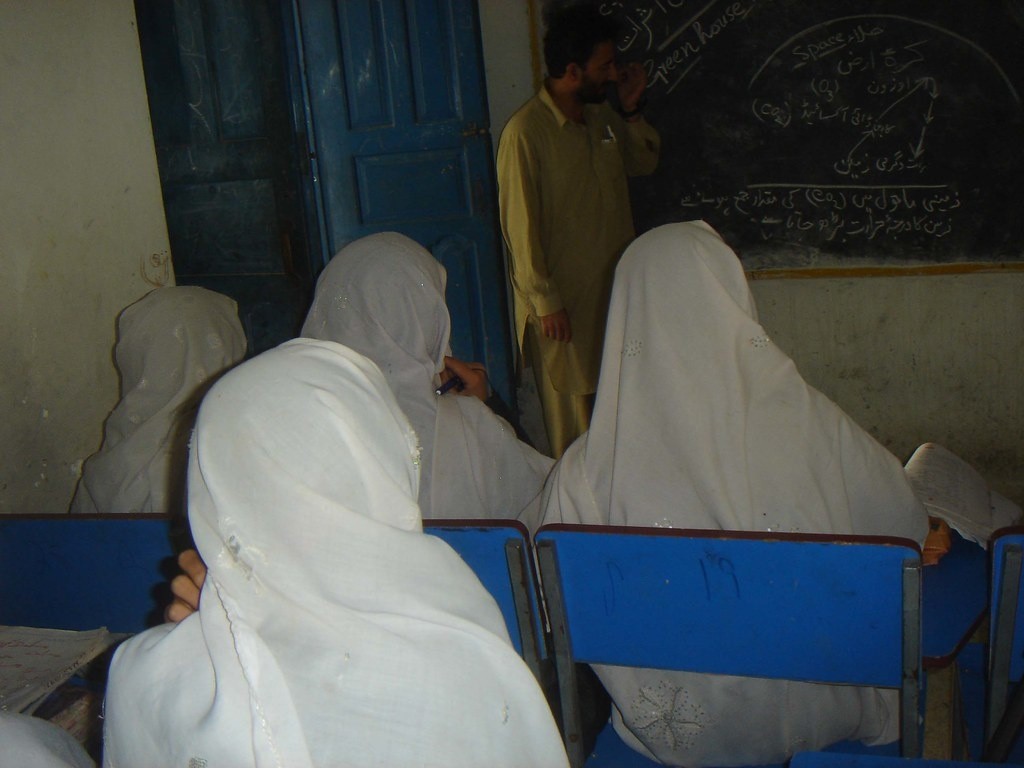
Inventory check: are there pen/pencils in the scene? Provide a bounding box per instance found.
[435,376,460,396]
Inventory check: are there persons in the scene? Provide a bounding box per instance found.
[298,231,556,519]
[70,286,251,513]
[548,217,951,766]
[100,338,566,766]
[494,8,662,462]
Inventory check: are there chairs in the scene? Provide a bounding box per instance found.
[2,513,203,636]
[416,519,557,697]
[534,514,990,768]
[952,526,1023,766]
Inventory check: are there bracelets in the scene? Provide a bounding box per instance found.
[621,109,638,118]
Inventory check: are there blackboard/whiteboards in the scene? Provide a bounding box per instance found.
[542,0,1023,264]
